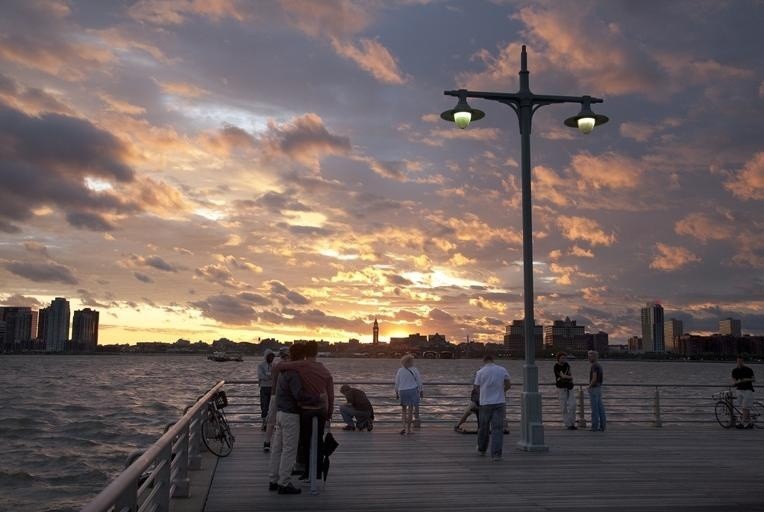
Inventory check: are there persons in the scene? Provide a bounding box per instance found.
[585,349,607,432]
[552,350,578,430]
[256,341,334,494]
[730,357,756,430]
[338,385,374,432]
[394,353,424,435]
[455,389,511,435]
[473,353,511,462]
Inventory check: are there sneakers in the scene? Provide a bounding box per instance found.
[478,450,486,456]
[277,483,302,496]
[342,425,356,432]
[267,482,280,491]
[263,440,271,450]
[491,456,503,465]
[735,422,754,430]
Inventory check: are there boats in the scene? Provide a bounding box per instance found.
[205,350,243,363]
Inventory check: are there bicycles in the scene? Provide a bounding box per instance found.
[708,390,763,430]
[202,396,235,457]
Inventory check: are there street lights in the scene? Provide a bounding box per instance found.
[436,43,612,456]
